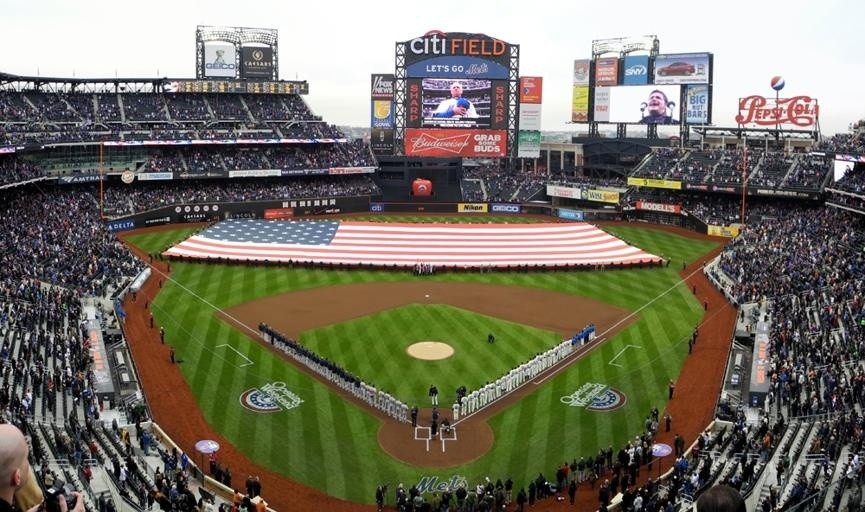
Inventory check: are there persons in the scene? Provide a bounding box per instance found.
[0,74,865,512]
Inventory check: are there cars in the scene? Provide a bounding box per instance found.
[657,62,696,77]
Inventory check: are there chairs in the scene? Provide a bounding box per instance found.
[692,418,865,511]
[18,421,159,511]
